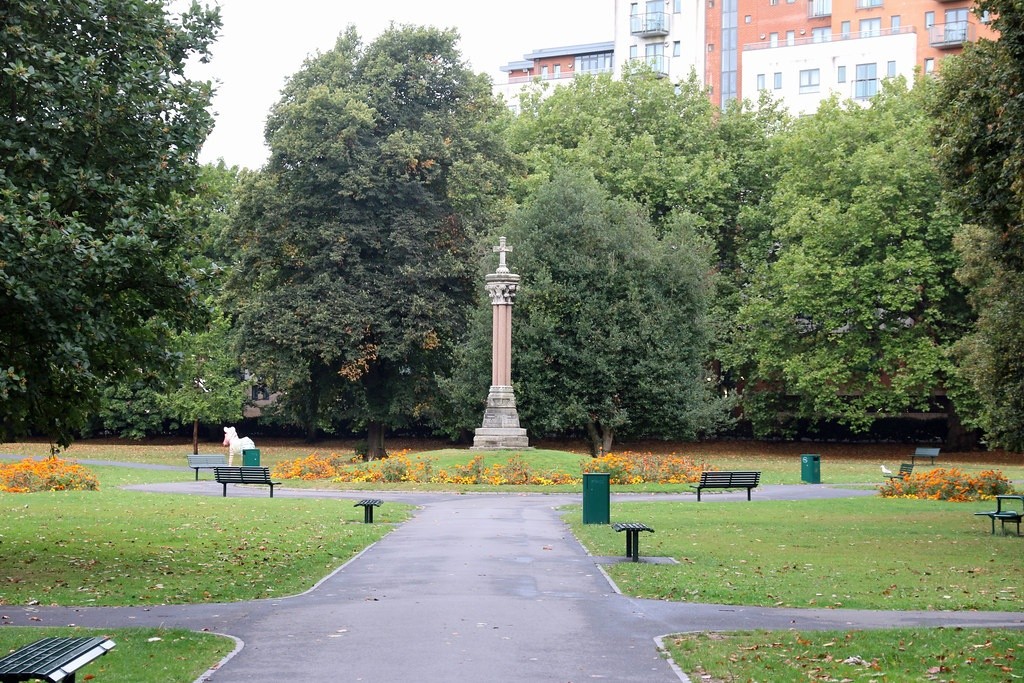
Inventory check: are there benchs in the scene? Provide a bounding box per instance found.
[909,448,940,465]
[214,466,282,498]
[354,499,384,523]
[974,494,1024,537]
[884,463,915,481]
[611,522,655,564]
[689,471,761,502]
[185,454,228,480]
[0,637,118,683]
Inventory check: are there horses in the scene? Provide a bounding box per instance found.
[222,426,255,467]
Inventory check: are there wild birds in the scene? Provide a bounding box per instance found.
[880,464,892,474]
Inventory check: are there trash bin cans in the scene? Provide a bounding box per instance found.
[583,472,612,525]
[800,453,822,486]
[242,448,261,467]
